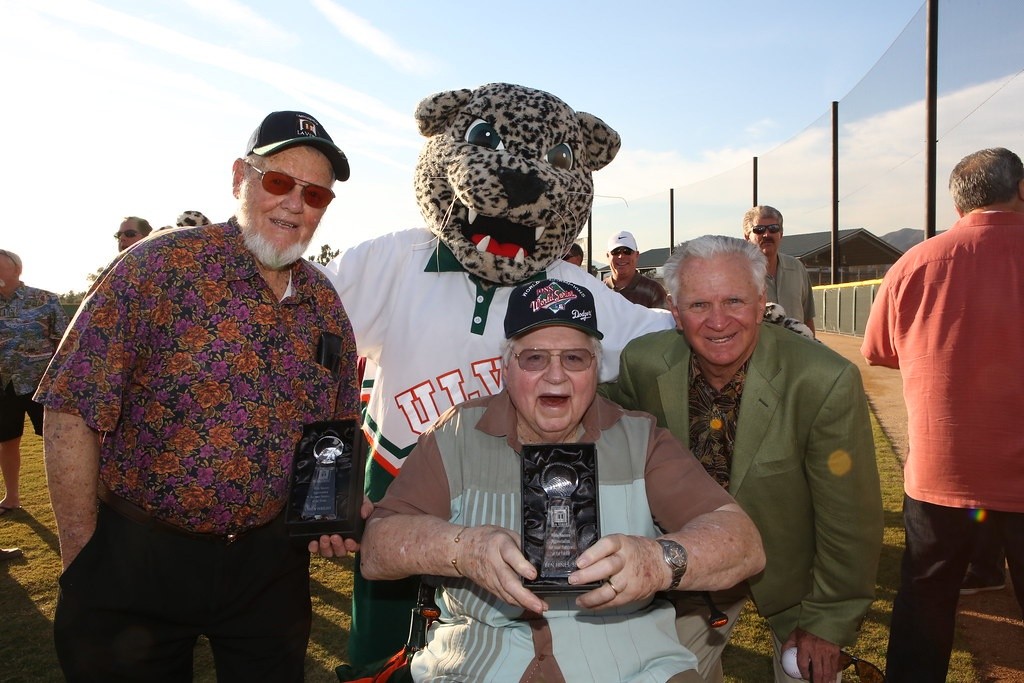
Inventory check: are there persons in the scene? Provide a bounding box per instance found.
[602,231,669,310]
[860,148,1024,683]
[563,243,583,267]
[744,206,816,338]
[113,217,153,253]
[361,280,767,683]
[596,235,884,683]
[0,248,70,516]
[31,112,374,683]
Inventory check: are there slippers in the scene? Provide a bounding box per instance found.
[0,504,23,517]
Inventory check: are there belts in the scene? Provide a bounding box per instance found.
[95,481,284,546]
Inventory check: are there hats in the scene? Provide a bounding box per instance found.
[609,230,637,252]
[245,111,350,182]
[504,279,604,342]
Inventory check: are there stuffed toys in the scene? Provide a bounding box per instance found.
[150,83,815,683]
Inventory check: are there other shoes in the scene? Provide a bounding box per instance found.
[959,573,1006,594]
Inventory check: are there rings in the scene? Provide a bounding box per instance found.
[607,580,618,595]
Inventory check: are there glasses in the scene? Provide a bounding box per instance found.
[751,224,781,234]
[612,248,632,256]
[0,249,16,265]
[508,346,596,371]
[114,230,149,240]
[836,650,885,683]
[245,161,336,209]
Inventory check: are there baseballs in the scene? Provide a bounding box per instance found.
[782,647,811,678]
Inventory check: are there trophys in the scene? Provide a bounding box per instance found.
[519,442,602,597]
[283,419,361,542]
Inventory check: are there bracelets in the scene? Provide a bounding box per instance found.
[452,527,469,575]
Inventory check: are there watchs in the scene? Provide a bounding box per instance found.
[656,539,687,592]
